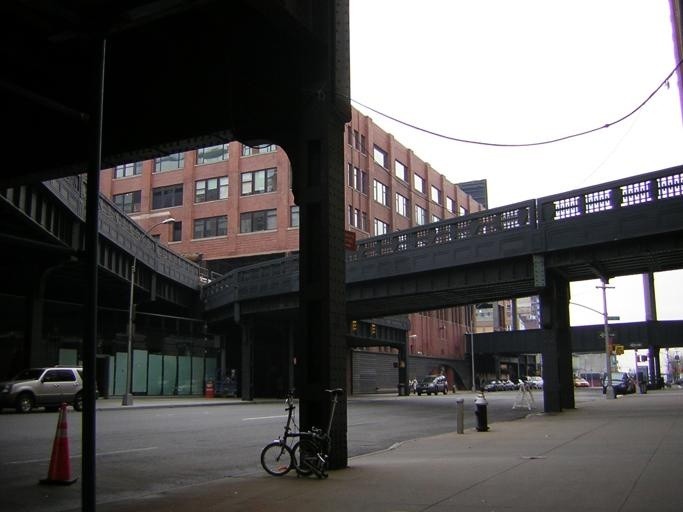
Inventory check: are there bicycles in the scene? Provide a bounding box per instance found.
[259,383,344,478]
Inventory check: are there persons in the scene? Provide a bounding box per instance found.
[411,377,418,394]
[409,377,412,392]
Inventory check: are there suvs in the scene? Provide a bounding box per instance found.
[417,374,448,395]
[1,366,100,414]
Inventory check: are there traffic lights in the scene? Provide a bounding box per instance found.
[353,320,358,332]
[371,324,376,335]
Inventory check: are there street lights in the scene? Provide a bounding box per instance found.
[629,343,642,395]
[464,330,475,390]
[121,217,177,406]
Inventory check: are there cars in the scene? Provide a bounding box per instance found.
[484,375,544,391]
[601,369,682,394]
[574,378,590,386]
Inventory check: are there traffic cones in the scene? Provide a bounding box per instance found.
[39,402,77,486]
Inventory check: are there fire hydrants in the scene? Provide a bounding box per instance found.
[474,391,491,433]
